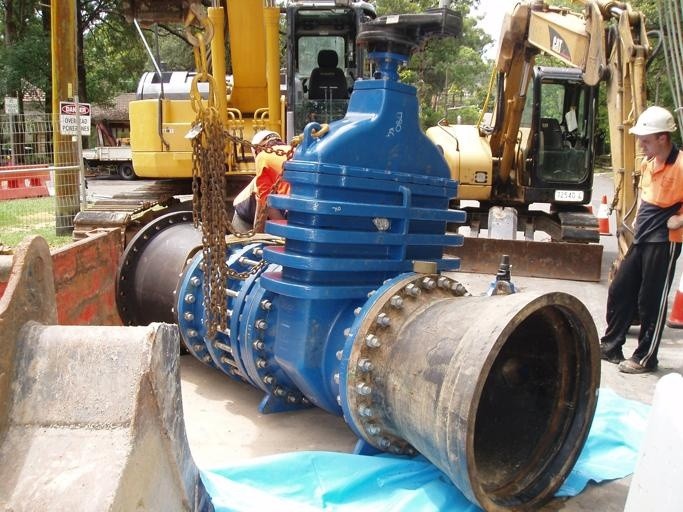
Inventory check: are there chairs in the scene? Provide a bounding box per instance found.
[308,49,347,99]
[539,118,585,182]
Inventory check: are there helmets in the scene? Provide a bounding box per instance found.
[628,106,678,136]
[250,130,281,159]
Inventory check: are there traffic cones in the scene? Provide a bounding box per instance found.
[667,271,683,329]
[597,192,612,236]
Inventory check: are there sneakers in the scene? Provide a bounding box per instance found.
[599,343,624,363]
[618,359,658,374]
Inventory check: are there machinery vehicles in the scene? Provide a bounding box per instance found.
[72,0,381,244]
[425,0,666,326]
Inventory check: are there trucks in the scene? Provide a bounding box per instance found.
[82,146,136,181]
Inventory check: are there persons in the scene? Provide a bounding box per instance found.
[248,130,290,234]
[229,177,286,233]
[599,105,683,376]
